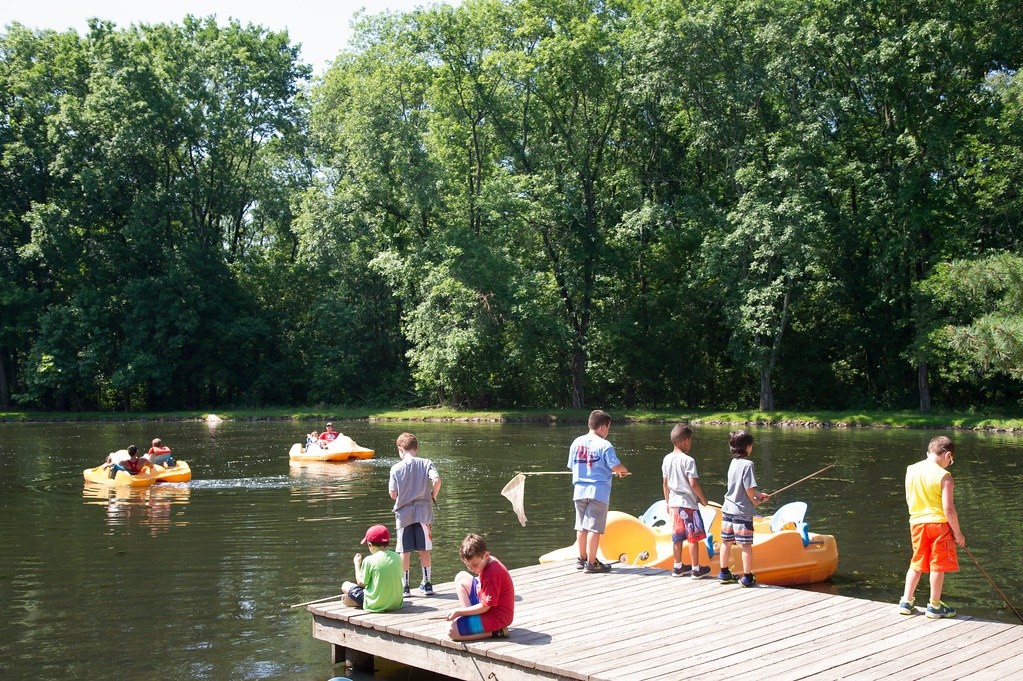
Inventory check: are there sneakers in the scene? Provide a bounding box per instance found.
[717,570,740,583]
[583,558,611,573]
[925,601,957,618]
[576,557,587,568]
[899,597,916,615]
[418,582,433,594]
[402,585,411,597]
[737,575,755,588]
[672,563,691,577]
[691,566,710,579]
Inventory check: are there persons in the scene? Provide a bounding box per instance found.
[340,522,406,613]
[149,438,171,465]
[566,410,627,572]
[308,431,319,443]
[319,422,338,442]
[444,530,514,641]
[388,432,442,598]
[898,437,966,618]
[718,430,771,588]
[661,423,713,580]
[103,444,154,475]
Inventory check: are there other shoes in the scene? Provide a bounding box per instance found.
[497,627,510,639]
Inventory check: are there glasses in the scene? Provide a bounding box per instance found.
[943,449,953,466]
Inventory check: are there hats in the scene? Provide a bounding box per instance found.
[360,524,389,545]
[326,423,333,427]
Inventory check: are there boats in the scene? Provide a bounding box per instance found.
[106,448,193,484]
[288,432,376,462]
[83,459,166,488]
[636,496,840,587]
[538,509,719,579]
